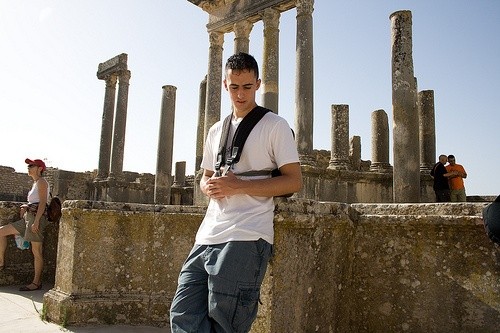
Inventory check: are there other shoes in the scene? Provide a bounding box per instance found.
[20,281,41,291]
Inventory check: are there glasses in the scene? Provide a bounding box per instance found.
[27,164,39,168]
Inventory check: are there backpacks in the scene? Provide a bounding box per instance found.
[36,176,61,223]
[215,106,295,197]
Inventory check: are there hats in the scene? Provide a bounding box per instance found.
[25,158,46,171]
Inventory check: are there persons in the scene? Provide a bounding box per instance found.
[445,155,467,202]
[0,157,51,290]
[170,53,303,333]
[430,155,457,202]
[482,195,500,243]
